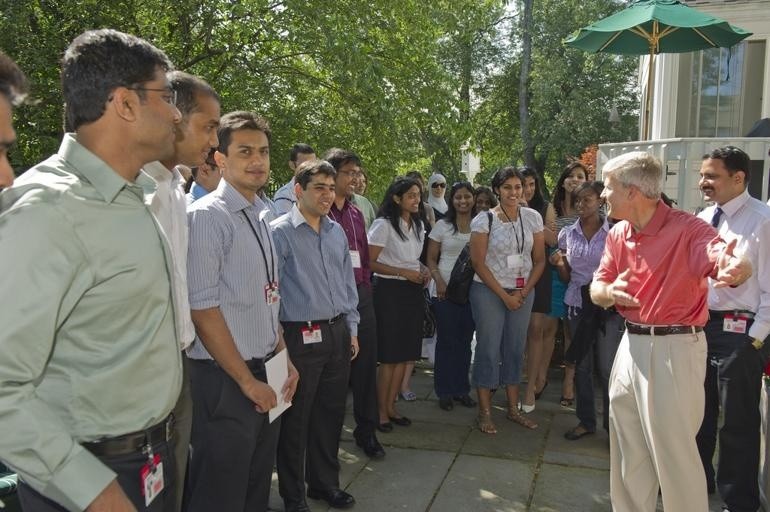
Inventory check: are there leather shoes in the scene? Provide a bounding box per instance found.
[452,391,477,408]
[353,426,386,461]
[387,413,414,427]
[559,395,575,408]
[564,421,596,441]
[278,493,312,512]
[305,477,356,509]
[375,414,394,435]
[439,392,454,412]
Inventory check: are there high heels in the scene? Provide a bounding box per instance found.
[513,398,536,413]
[532,378,550,401]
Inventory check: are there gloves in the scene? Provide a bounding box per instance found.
[717,341,758,384]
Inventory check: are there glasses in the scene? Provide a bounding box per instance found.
[337,167,364,178]
[430,182,447,188]
[104,85,181,108]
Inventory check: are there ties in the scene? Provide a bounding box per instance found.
[707,206,725,228]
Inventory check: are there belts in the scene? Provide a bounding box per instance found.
[703,309,759,324]
[182,349,277,370]
[279,313,343,329]
[79,412,177,460]
[620,319,707,336]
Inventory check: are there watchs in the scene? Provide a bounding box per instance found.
[750,338,766,351]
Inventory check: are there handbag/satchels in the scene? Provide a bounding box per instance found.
[442,241,477,307]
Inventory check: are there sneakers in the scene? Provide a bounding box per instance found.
[393,389,417,402]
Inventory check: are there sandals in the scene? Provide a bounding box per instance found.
[505,411,539,430]
[474,408,499,434]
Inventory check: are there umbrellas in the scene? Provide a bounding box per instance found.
[562,0,754,141]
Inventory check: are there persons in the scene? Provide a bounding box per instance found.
[137,71,616,512]
[0,31,223,512]
[696,146,770,512]
[0,50,28,197]
[588,152,752,512]
